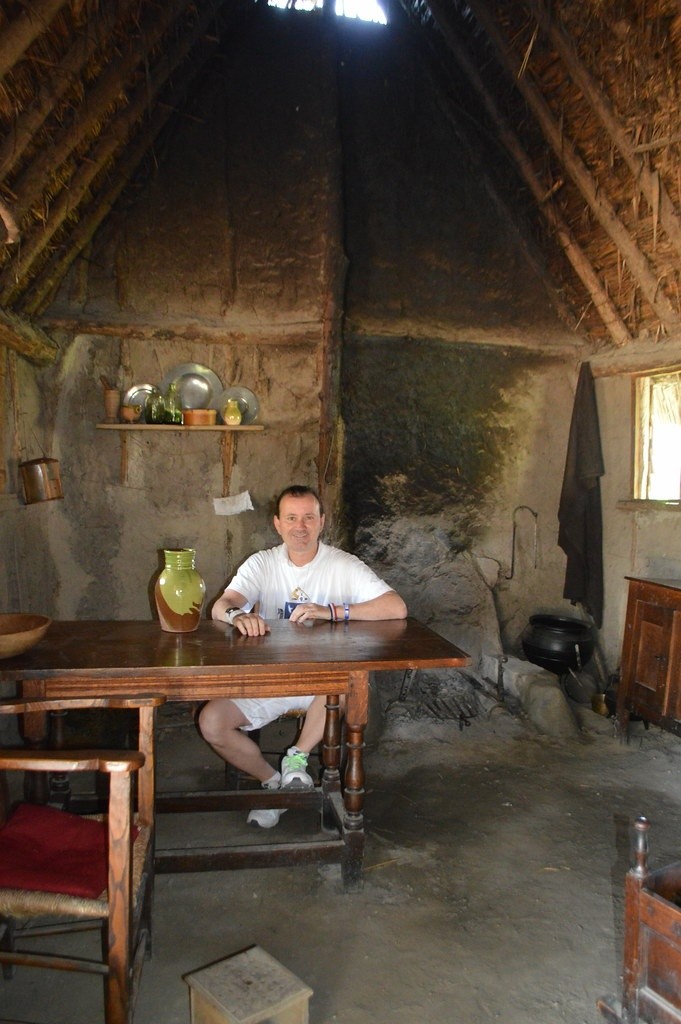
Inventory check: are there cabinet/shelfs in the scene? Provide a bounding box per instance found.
[611,576,681,747]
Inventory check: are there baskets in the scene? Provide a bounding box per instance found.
[0,613,54,660]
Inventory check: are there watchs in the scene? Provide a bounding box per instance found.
[225,607,240,619]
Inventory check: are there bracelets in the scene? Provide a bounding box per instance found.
[328,603,337,621]
[343,602,349,620]
[230,610,246,624]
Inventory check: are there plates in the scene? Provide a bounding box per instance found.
[219,387,259,424]
[123,383,161,423]
[161,362,223,413]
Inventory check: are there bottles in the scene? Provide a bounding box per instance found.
[226,402,241,424]
[154,545,208,633]
[145,387,165,423]
[164,383,183,424]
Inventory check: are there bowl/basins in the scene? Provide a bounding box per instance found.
[0,612,50,661]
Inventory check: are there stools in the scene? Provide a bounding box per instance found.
[223,708,345,793]
[184,945,314,1024]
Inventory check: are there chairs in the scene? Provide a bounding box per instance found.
[0,692,168,1023]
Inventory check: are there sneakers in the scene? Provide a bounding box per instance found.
[278,747,315,792]
[246,775,289,829]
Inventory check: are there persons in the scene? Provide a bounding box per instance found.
[198,485,408,828]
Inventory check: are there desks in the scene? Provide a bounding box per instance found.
[0,618,473,893]
